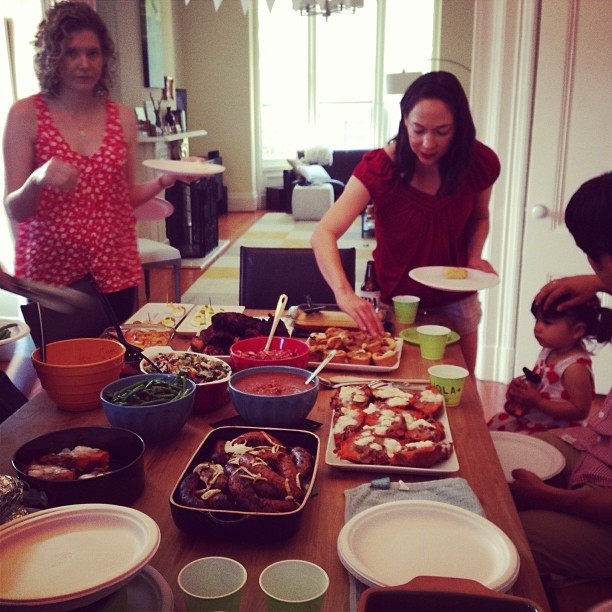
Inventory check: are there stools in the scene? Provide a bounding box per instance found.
[292,183,334,221]
[136,236,183,303]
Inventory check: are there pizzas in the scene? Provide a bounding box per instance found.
[110,330,172,349]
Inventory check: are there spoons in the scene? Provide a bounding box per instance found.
[90,281,144,362]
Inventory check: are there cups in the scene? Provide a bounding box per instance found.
[257,551,329,608]
[429,365,470,407]
[418,323,450,361]
[390,294,421,327]
[177,552,249,606]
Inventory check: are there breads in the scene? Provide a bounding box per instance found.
[444,267,468,280]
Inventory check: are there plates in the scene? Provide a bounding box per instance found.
[491,425,567,480]
[0,500,162,605]
[142,155,225,181]
[407,264,502,295]
[308,328,406,373]
[100,324,176,348]
[170,425,321,543]
[400,326,462,346]
[116,301,194,331]
[287,303,391,331]
[173,302,245,336]
[338,498,520,593]
[186,313,295,356]
[326,386,462,475]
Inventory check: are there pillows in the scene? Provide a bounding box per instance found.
[287,158,310,179]
[298,165,331,182]
[305,136,340,166]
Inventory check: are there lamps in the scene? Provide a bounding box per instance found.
[293,0,363,23]
[385,69,422,94]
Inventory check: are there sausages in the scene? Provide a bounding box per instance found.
[177,432,313,512]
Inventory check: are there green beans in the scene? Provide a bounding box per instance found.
[104,375,191,406]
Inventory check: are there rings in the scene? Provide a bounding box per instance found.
[550,278,558,287]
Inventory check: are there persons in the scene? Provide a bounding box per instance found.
[486,290,612,436]
[509,170,610,586]
[310,70,500,378]
[4,1,213,348]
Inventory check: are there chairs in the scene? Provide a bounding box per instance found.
[238,245,356,310]
[20,271,111,349]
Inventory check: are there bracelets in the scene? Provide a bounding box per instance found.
[158,175,174,190]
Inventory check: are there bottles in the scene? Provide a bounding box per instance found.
[361,260,383,334]
[503,365,543,418]
[166,106,176,129]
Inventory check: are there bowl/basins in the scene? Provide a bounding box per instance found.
[0,317,31,362]
[139,351,233,412]
[31,332,126,411]
[227,335,311,363]
[99,372,198,445]
[228,363,325,428]
[11,425,146,509]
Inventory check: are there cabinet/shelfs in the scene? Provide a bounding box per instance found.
[135,130,207,242]
[266,186,284,210]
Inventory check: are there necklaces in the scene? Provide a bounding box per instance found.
[57,95,94,138]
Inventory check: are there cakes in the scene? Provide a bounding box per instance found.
[307,327,399,366]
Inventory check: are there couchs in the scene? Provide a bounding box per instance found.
[285,149,373,215]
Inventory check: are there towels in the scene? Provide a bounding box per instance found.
[344,477,514,612]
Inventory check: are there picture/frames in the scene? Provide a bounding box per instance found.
[140,0,164,90]
